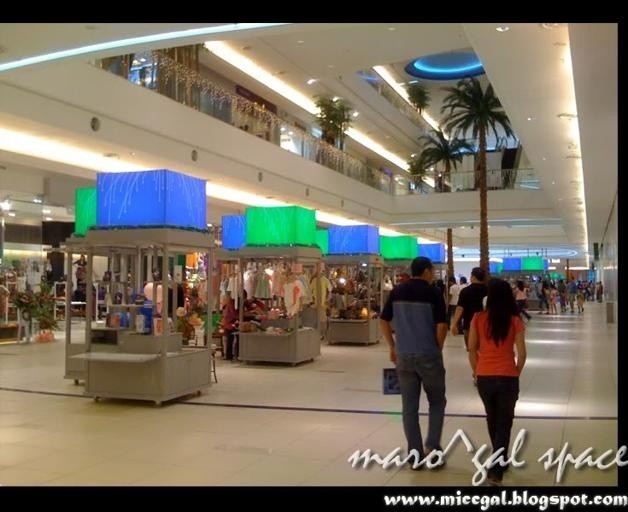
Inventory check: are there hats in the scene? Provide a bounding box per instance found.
[176,307,187,316]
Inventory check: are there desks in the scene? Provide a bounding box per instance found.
[54,301,87,324]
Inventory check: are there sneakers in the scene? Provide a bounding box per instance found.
[562,308,584,313]
[528,316,531,320]
[538,312,557,314]
[411,448,445,471]
[487,463,509,485]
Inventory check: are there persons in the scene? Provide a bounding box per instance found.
[467,279,527,485]
[222,289,250,361]
[72,280,109,313]
[433,267,603,335]
[381,256,451,470]
[71,254,88,286]
[382,273,393,309]
[451,264,490,388]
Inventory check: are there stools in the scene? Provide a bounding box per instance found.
[202,334,225,357]
[211,349,218,383]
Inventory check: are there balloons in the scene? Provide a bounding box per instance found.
[144,283,164,304]
